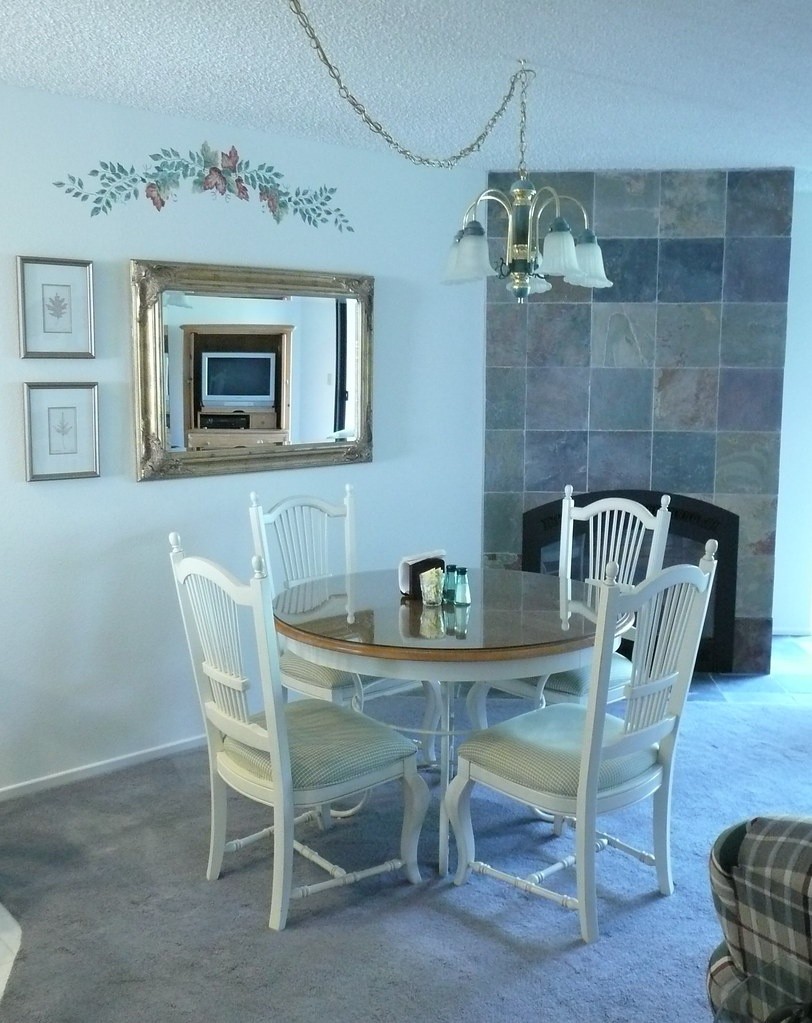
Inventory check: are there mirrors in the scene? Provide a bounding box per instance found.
[129,258,375,482]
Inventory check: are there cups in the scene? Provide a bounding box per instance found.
[419,578,444,606]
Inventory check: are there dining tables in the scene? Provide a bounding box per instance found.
[268,566,636,881]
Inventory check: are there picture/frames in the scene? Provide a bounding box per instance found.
[24,381,101,482]
[16,254,95,360]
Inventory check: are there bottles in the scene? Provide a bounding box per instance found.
[454,567,472,605]
[444,564,458,602]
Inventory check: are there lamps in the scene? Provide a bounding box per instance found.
[432,65,618,305]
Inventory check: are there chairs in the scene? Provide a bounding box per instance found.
[441,537,722,948]
[464,482,673,735]
[168,530,433,932]
[246,478,443,771]
